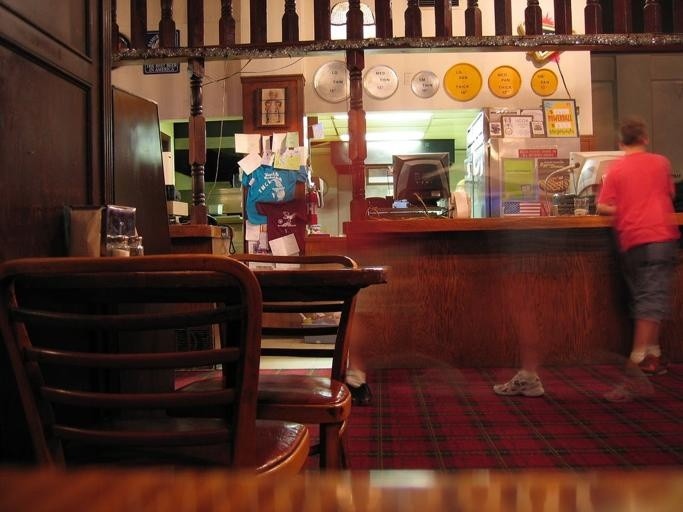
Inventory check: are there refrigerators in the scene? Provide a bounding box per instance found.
[464,137,580,218]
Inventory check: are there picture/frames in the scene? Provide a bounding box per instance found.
[535,158,569,215]
[520,110,544,122]
[488,108,521,138]
[500,115,533,138]
[515,13,576,70]
[529,121,545,138]
[542,98,578,138]
[261,88,284,126]
[143,30,180,74]
[498,157,540,218]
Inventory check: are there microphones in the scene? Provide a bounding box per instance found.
[546,162,580,216]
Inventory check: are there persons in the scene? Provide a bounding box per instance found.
[491,263,656,406]
[344,287,374,406]
[594,119,683,377]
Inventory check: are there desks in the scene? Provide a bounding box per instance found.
[17,267,388,474]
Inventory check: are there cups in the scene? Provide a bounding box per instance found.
[573,198,589,216]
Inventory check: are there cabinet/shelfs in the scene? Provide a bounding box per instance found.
[239,74,309,235]
[419,138,455,165]
[241,235,347,339]
[330,140,352,174]
[464,108,580,219]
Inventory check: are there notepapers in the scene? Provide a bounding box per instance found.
[308,116,318,124]
[308,127,313,138]
[312,124,325,139]
[233,131,307,272]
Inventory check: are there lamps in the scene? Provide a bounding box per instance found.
[330,2,375,40]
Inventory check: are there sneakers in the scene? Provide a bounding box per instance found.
[626,359,670,378]
[345,382,373,406]
[603,380,658,404]
[658,355,673,367]
[493,374,545,398]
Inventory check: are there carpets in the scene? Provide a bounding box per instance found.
[174,366,682,471]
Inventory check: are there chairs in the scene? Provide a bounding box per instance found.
[174,253,359,474]
[0,254,310,476]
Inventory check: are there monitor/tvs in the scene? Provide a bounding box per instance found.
[392,152,452,207]
[570,152,625,196]
